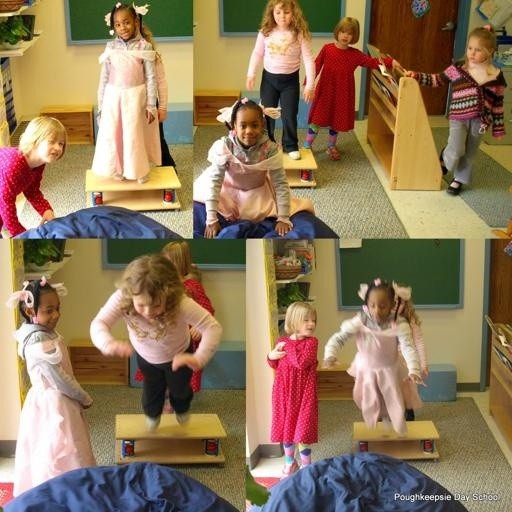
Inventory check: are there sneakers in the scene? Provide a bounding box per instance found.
[327,146,339,160]
[288,150,300,159]
[447,180,462,194]
[280,459,298,480]
[440,148,448,174]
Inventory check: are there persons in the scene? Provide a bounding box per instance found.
[0,115,67,240]
[246,0,316,160]
[134,239,216,413]
[266,301,319,480]
[91,2,163,183]
[403,24,507,195]
[141,26,178,174]
[5,275,96,498]
[202,97,293,239]
[321,277,427,438]
[87,254,224,430]
[392,282,430,421]
[300,17,403,162]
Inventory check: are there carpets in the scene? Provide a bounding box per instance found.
[431,126,512,228]
[315,386,512,511]
[195,123,409,238]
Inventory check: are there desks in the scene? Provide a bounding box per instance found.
[114,413,226,468]
[281,147,317,191]
[350,421,440,463]
[84,167,182,215]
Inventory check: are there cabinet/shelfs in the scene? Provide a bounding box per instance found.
[40,105,94,145]
[265,239,315,351]
[67,343,128,384]
[194,90,241,127]
[365,62,443,190]
[316,368,354,402]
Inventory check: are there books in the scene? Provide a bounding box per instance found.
[500,353,511,369]
[388,75,398,87]
[381,86,392,104]
